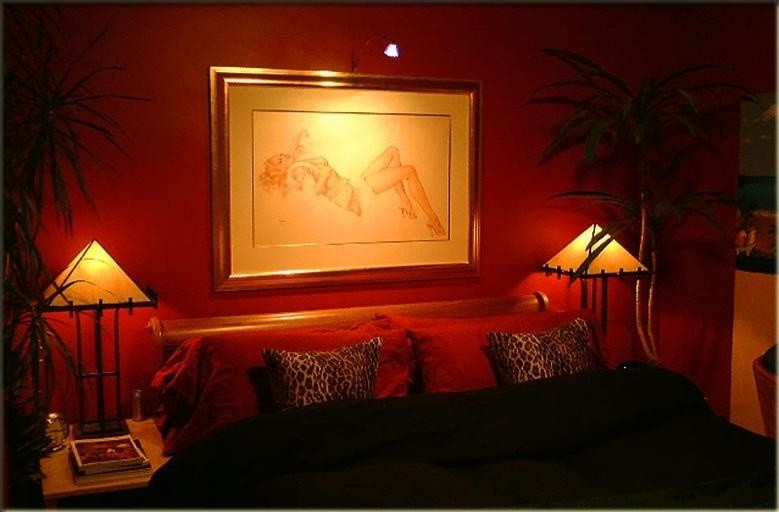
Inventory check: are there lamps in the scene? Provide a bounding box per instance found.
[541,220,650,337]
[37,239,154,440]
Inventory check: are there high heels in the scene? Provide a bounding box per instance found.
[426,216,446,236]
[397,199,417,218]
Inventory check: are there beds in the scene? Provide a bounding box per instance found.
[146,294,776,506]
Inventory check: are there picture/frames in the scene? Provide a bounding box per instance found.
[209,65,483,293]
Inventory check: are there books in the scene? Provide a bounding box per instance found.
[68,434,153,485]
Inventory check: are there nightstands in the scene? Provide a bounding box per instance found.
[39,419,175,509]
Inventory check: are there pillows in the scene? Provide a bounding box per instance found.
[485,317,602,386]
[266,339,381,409]
[376,304,608,397]
[152,320,417,454]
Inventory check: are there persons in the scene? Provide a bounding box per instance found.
[258,145,447,238]
